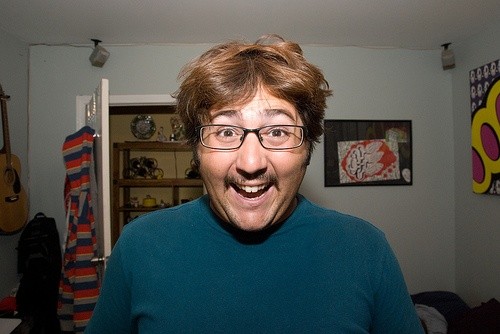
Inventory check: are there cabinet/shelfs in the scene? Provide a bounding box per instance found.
[111,141,207,248]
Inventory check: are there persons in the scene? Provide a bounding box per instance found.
[83,42,426,334]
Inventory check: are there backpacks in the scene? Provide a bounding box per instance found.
[16,212,62,276]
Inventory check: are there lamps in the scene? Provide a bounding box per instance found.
[441,42,456,70]
[89,37,111,69]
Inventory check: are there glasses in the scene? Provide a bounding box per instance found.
[191,122,310,149]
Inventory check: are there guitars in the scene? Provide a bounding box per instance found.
[0,86,31,234]
